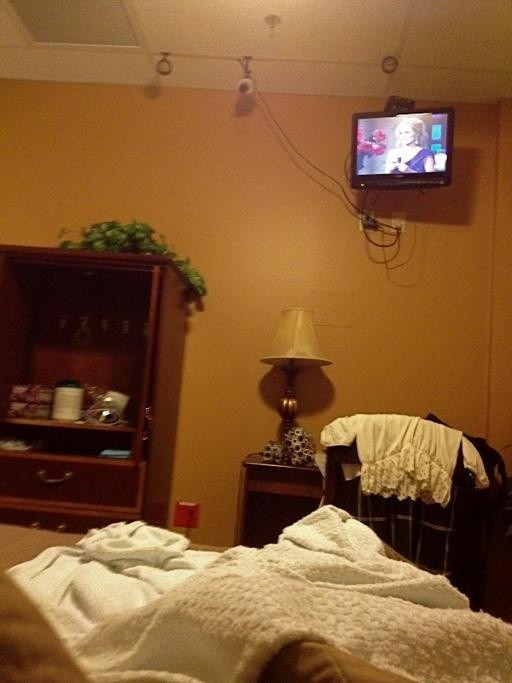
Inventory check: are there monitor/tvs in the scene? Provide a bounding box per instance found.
[348,95,455,189]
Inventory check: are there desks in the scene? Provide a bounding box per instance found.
[234,452,325,546]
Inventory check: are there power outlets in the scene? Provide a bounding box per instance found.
[391,210,407,233]
[357,208,376,233]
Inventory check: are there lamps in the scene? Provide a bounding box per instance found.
[259,305,333,441]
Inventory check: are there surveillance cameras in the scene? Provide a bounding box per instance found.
[236,55,255,98]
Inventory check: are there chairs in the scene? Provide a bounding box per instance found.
[324,412,463,598]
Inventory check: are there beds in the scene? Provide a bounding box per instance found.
[0,522,511,681]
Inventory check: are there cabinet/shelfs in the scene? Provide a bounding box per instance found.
[1,242,197,533]
